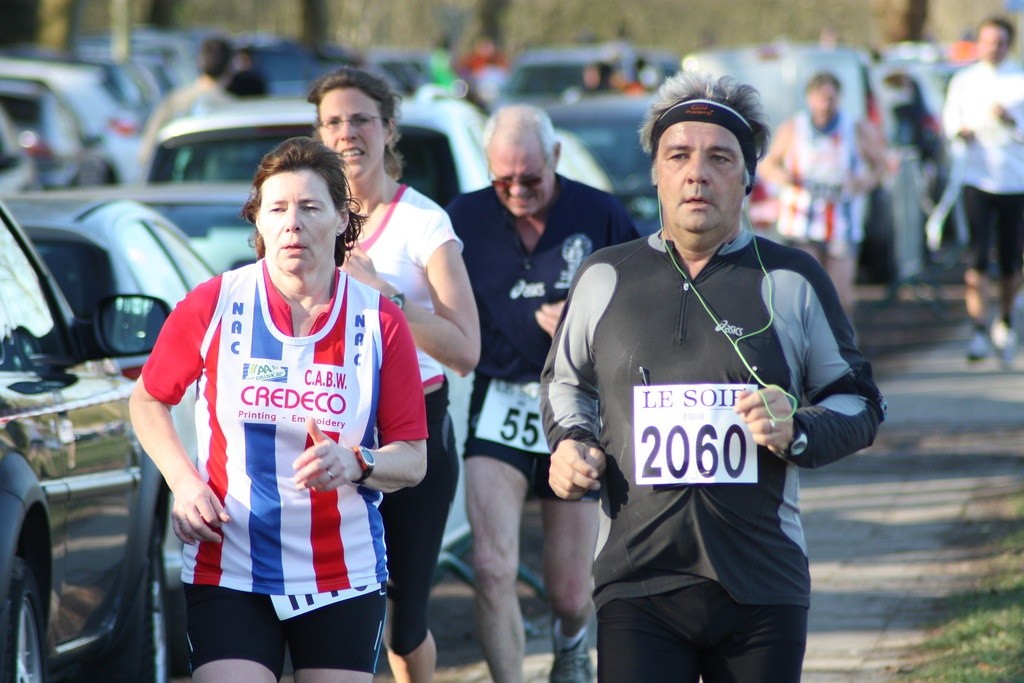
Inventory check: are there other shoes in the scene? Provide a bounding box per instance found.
[991,317,1016,356]
[967,325,988,359]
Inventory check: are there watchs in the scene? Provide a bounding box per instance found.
[352,446,375,485]
[390,293,406,309]
[783,420,808,458]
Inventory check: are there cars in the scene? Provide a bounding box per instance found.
[0,197,194,683]
[0,27,1022,612]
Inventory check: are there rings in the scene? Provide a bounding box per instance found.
[326,470,334,481]
[769,419,775,434]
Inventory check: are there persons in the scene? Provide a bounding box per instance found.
[444,105,641,683]
[539,70,889,683]
[58,411,77,469]
[308,70,482,683]
[226,48,267,100]
[29,416,56,473]
[432,28,509,102]
[942,18,1024,362]
[131,135,430,683]
[760,76,887,318]
[138,39,235,167]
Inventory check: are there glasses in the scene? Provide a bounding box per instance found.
[488,154,548,187]
[319,116,389,129]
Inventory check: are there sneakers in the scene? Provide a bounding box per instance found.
[549,627,595,683]
